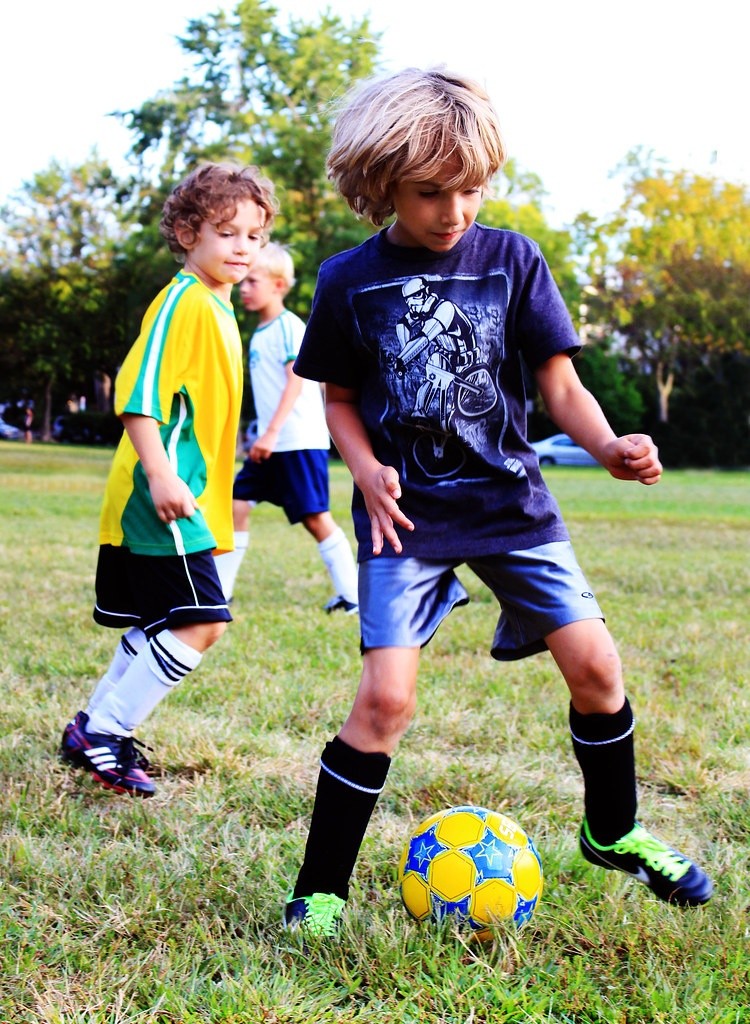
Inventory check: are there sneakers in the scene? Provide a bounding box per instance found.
[61,711,157,797]
[282,891,347,952]
[577,812,712,908]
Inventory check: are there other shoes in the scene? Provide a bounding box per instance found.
[225,596,234,609]
[325,595,359,615]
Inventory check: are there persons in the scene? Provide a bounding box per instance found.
[65,163,272,796]
[213,245,368,618]
[284,70,714,937]
[26,408,34,442]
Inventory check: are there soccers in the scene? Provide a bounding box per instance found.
[396,804,546,947]
